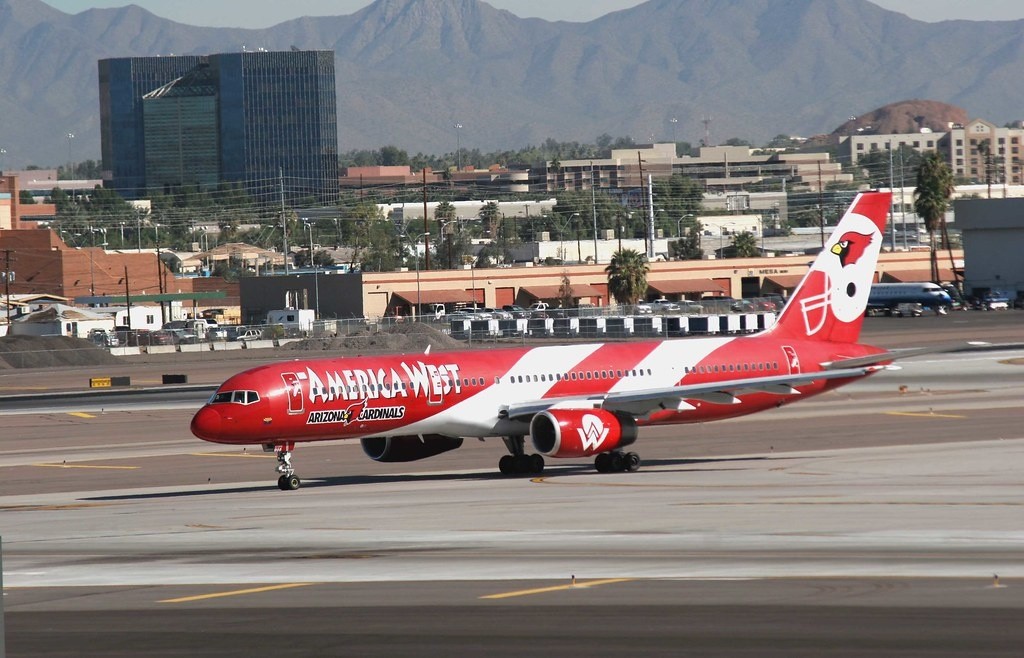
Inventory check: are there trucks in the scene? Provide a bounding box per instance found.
[427,303,459,321]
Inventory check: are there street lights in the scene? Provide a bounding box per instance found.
[560,212,582,266]
[154,223,160,248]
[66,133,75,181]
[414,231,432,322]
[630,206,665,261]
[119,221,126,246]
[440,220,458,244]
[720,222,736,259]
[678,213,694,238]
[453,124,466,172]
[304,223,323,269]
[531,214,547,257]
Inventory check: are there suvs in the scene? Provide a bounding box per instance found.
[484,307,513,321]
[761,293,786,310]
[459,307,492,321]
[502,305,531,318]
[701,295,744,313]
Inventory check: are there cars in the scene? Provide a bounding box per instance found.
[85,316,263,342]
[735,298,758,312]
[677,300,708,313]
[752,297,778,313]
[624,300,653,316]
[527,301,566,318]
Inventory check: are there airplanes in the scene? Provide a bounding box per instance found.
[865,283,950,319]
[189,190,903,491]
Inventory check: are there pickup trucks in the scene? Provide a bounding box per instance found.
[649,298,680,314]
